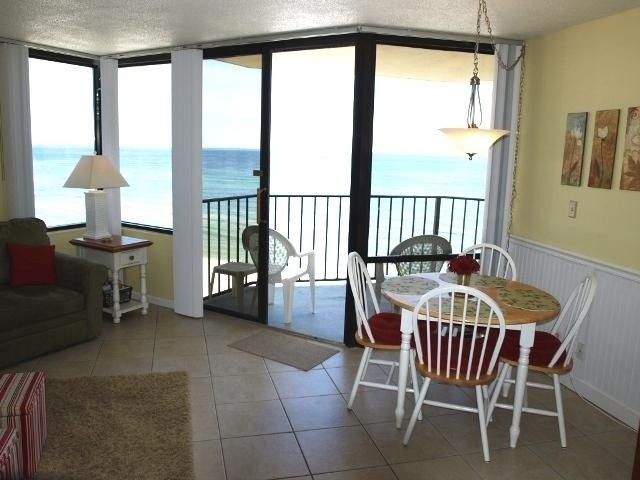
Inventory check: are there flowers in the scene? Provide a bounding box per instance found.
[447,253,483,280]
[564,127,582,186]
[597,127,608,188]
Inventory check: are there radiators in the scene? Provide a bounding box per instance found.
[504,234,640,432]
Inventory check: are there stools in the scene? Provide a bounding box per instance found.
[0,367,47,480]
[0,427,24,480]
[207,261,258,314]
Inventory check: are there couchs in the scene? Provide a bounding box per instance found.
[0,218,108,371]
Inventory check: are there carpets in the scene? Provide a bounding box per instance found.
[227,331,340,372]
[31,370,195,479]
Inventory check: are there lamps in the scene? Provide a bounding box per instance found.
[435,1,526,282]
[62,154,129,240]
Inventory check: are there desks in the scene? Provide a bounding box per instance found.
[380,271,563,449]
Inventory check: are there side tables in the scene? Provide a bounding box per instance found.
[69,235,153,324]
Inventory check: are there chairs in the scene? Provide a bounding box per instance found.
[346,251,425,423]
[484,275,597,449]
[442,243,518,279]
[390,235,452,276]
[401,284,504,463]
[242,225,317,324]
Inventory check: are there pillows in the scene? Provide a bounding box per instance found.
[6,241,56,288]
